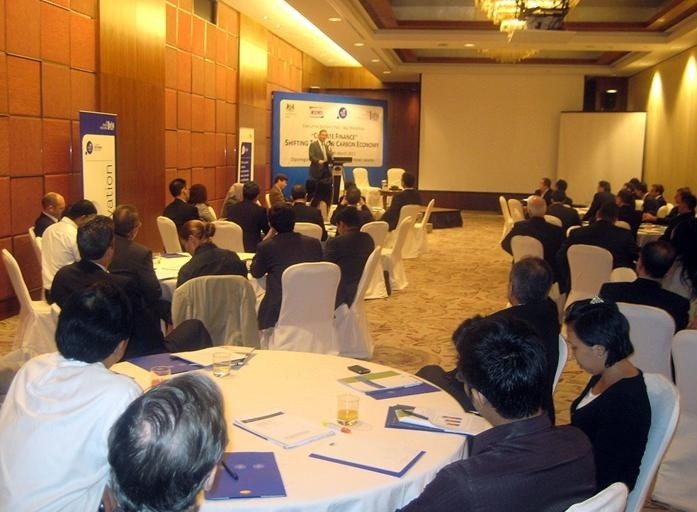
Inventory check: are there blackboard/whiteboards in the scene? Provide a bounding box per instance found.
[556,111,648,205]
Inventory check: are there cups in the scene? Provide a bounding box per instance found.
[151,364,171,387]
[210,350,232,379]
[336,393,361,426]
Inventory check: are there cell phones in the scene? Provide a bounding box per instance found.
[347,364,370,375]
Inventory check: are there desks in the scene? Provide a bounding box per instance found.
[103,344,471,512]
[379,188,416,209]
[307,201,387,238]
[571,199,669,248]
[153,253,268,337]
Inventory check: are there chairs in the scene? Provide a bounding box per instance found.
[265,192,272,209]
[382,216,413,292]
[560,245,614,342]
[171,276,261,351]
[2,249,57,356]
[396,205,420,260]
[336,245,382,361]
[415,199,436,246]
[360,221,390,300]
[260,261,342,355]
[386,168,406,207]
[293,221,322,243]
[613,302,675,379]
[657,202,674,217]
[651,329,696,511]
[552,334,679,512]
[352,167,382,206]
[156,216,183,254]
[499,195,527,243]
[208,219,245,253]
[504,234,561,309]
[27,226,45,301]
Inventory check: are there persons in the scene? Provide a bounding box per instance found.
[564,295,652,494]
[39,200,97,305]
[395,315,597,512]
[34,192,65,237]
[107,372,229,511]
[379,172,423,232]
[222,174,328,253]
[176,219,248,286]
[250,203,323,330]
[308,130,332,180]
[323,208,375,308]
[49,217,214,357]
[414,257,560,411]
[500,178,697,334]
[107,206,172,325]
[0,279,144,511]
[163,179,217,231]
[330,182,375,236]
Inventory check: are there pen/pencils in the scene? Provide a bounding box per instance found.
[221,460,238,481]
[404,410,428,421]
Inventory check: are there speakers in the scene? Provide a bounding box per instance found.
[583,74,628,111]
